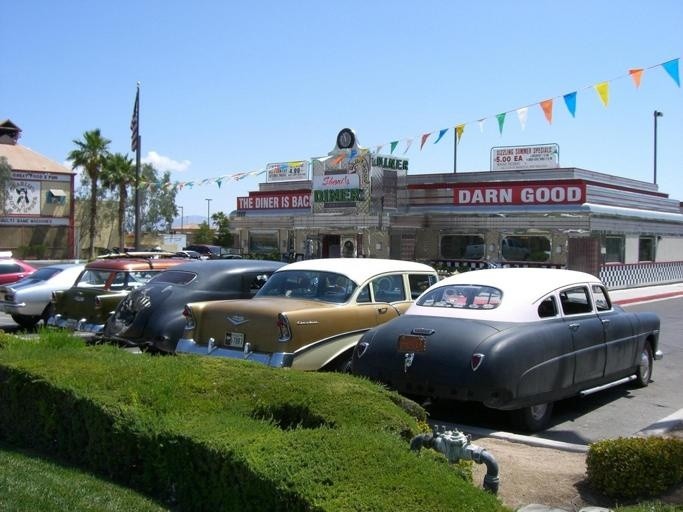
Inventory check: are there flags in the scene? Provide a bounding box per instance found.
[130,88,140,150]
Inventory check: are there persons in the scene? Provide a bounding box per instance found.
[17,188,28,208]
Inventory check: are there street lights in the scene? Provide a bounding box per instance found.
[204,198,211,227]
[653,110,664,184]
[177,206,184,230]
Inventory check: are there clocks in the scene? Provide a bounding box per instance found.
[336,128,355,150]
